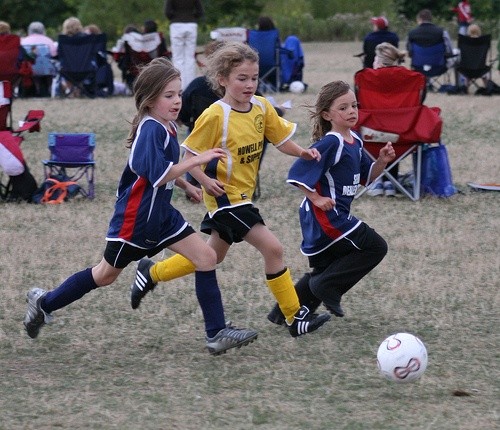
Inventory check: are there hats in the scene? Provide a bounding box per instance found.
[370,17,389,30]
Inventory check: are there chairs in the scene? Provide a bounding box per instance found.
[453,33,499,92]
[0,30,171,198]
[194,26,247,73]
[411,42,457,90]
[248,30,294,95]
[354,66,443,200]
[353,45,397,69]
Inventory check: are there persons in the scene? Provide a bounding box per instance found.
[22,56,259,357]
[166,0,204,92]
[0,17,172,99]
[352,0,490,95]
[260,15,294,58]
[131,41,332,339]
[176,41,284,203]
[267,80,396,328]
[353,43,408,199]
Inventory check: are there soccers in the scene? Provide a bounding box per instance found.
[289,81,305,94]
[376,333,429,384]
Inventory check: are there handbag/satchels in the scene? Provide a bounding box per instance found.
[412,140,457,196]
[117,45,132,73]
[51,74,66,99]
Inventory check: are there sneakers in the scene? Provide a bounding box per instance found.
[206,322,258,356]
[131,259,155,310]
[23,288,54,339]
[309,276,345,317]
[367,181,383,196]
[384,181,395,197]
[267,303,286,325]
[284,305,330,337]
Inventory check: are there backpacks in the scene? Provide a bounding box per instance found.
[83,50,113,98]
[278,36,305,82]
[33,174,80,203]
